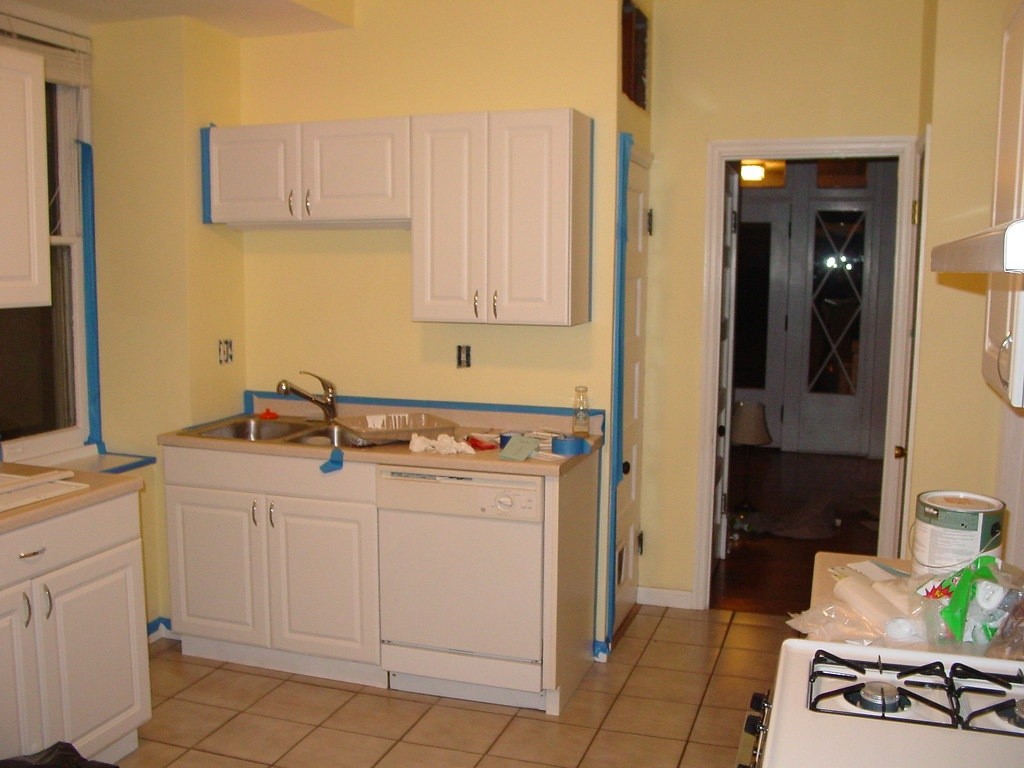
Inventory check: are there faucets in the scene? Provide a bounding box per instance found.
[276,370,338,423]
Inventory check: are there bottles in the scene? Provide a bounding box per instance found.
[572,386,590,438]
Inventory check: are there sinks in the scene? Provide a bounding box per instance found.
[277,423,377,451]
[176,416,320,443]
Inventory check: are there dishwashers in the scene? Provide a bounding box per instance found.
[375,464,544,693]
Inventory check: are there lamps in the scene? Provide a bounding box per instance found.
[732,399,773,514]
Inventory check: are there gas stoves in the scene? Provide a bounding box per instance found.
[772,649,1024,740]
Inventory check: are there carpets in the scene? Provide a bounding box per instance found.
[729,480,840,539]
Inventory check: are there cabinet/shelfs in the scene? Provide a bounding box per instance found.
[411,108,594,326]
[209,116,411,230]
[982,9,1024,409]
[162,445,382,665]
[0,46,54,308]
[0,491,152,768]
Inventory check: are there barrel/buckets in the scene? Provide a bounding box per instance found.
[908,491,1006,586]
